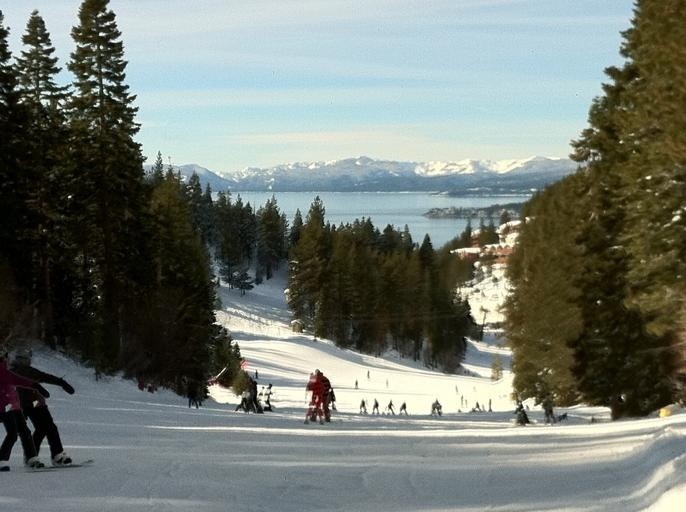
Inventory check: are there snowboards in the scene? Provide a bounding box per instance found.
[47,459,93,469]
[14,468,53,473]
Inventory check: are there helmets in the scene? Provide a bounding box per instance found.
[13,348,32,359]
[0,344,8,357]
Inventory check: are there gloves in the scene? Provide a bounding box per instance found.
[57,377,75,394]
[36,384,50,399]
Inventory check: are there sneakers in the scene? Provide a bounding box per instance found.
[24,460,45,468]
[0,462,11,472]
[52,455,72,464]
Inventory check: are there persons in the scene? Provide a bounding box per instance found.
[431,400,442,417]
[138,380,158,394]
[305,369,336,423]
[186,378,202,409]
[372,398,379,414]
[234,369,274,414]
[360,400,367,414]
[541,393,558,423]
[387,400,395,415]
[10,346,75,466]
[0,349,51,471]
[400,402,408,415]
